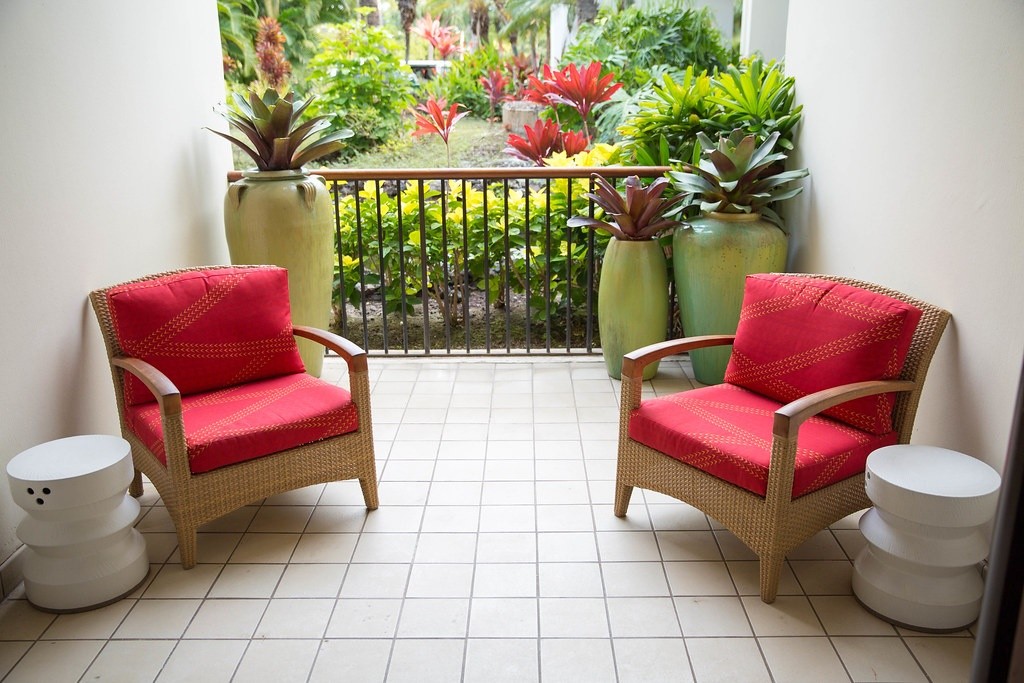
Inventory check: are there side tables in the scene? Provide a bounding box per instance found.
[851,444,1003,633]
[6,434,150,613]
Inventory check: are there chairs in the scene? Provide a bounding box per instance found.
[88,264,379,569]
[613,274,952,604]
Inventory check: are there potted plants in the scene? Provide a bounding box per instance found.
[199,87,354,377]
[566,172,693,381]
[663,128,810,384]
